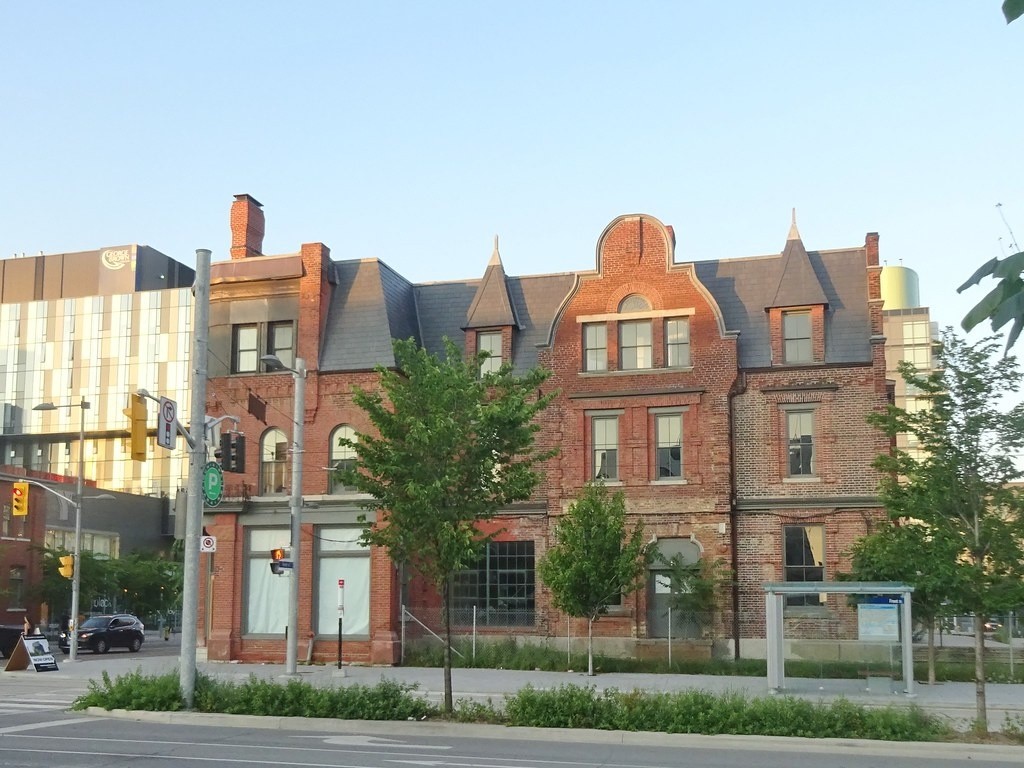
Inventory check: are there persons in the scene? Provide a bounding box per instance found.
[21,614,36,636]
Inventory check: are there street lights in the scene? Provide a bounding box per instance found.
[32,396,85,663]
[260,351,306,674]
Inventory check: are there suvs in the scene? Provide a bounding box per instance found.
[58,614,145,655]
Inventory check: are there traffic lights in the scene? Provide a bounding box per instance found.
[231,436,246,473]
[122,393,147,462]
[58,556,72,578]
[213,432,232,473]
[12,482,29,516]
[273,548,283,561]
